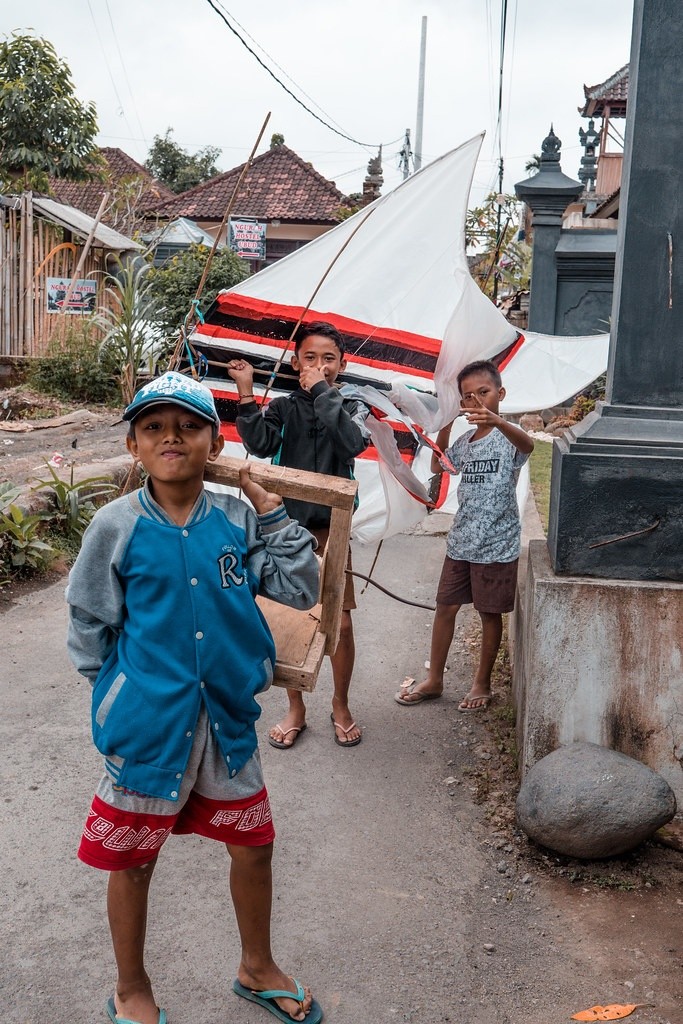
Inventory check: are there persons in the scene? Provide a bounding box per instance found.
[66,373,322,1024]
[394,360,534,713]
[228,321,370,746]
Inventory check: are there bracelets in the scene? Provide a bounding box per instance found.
[443,427,451,432]
[239,393,254,398]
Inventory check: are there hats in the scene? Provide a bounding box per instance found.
[122,371,222,433]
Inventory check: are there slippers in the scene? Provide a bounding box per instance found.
[458,687,495,713]
[395,680,445,706]
[269,715,308,748]
[330,709,361,747]
[233,969,324,1024]
[107,993,168,1024]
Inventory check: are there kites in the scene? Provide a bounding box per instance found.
[166,131,610,547]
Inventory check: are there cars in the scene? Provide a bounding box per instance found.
[96,316,187,380]
